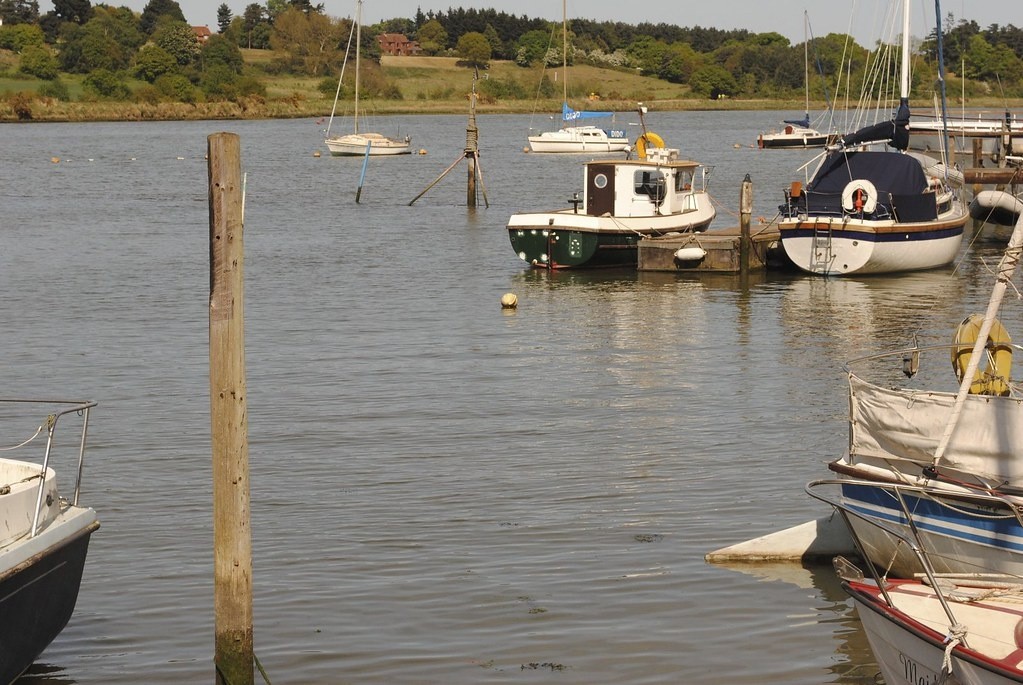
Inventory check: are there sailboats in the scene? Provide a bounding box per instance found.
[322,1,415,156]
[753,8,836,149]
[525,0,632,153]
[772,1,970,280]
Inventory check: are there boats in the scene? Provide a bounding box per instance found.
[707,214,1022,588]
[904,114,1023,154]
[806,477,1022,684]
[0,400,101,685]
[506,102,719,275]
